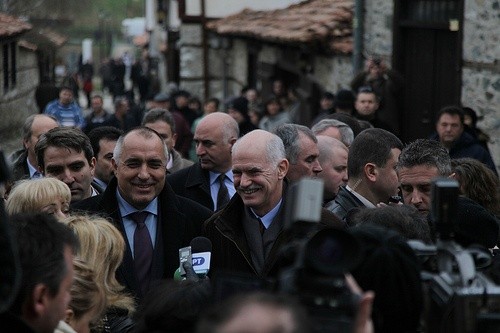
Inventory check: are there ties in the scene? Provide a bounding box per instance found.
[126,211,154,280]
[33,172,40,178]
[216,175,230,213]
[257,218,264,237]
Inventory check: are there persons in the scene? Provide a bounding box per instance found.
[282,59,500,333]
[201,130,342,287]
[0,50,261,333]
[201,287,313,333]
[70,126,214,300]
[260,94,292,134]
[271,80,285,94]
[274,123,322,184]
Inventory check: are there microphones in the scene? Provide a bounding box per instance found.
[190,236,213,279]
